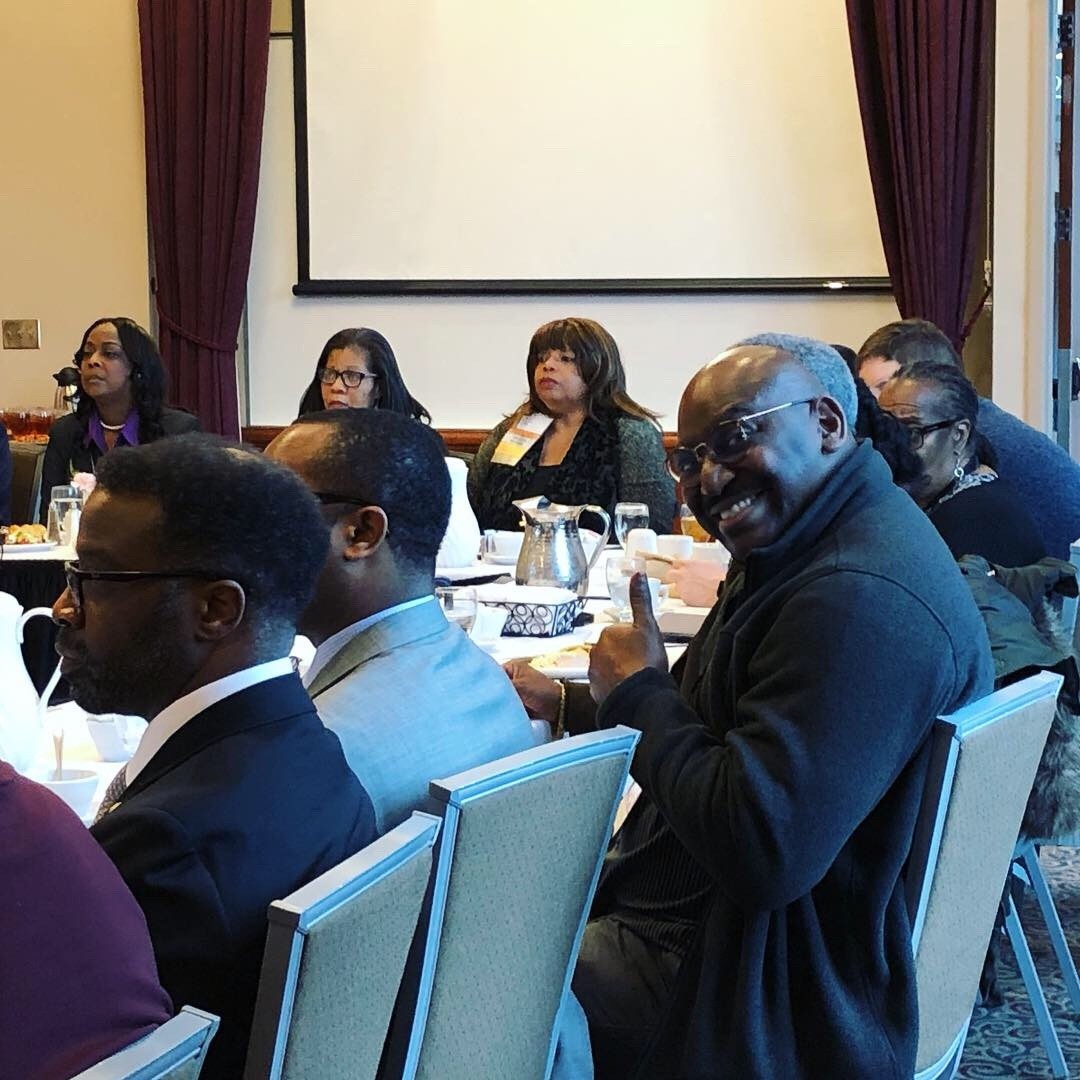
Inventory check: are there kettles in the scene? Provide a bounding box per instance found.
[52,367,81,413]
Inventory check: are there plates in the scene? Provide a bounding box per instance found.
[0,542,57,553]
[485,554,517,564]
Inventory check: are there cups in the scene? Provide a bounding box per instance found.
[615,502,649,549]
[606,558,647,610]
[657,535,692,560]
[680,504,711,543]
[437,586,476,635]
[0,407,55,445]
[647,578,668,610]
[692,543,721,564]
[50,485,83,525]
[625,529,657,561]
[33,769,100,817]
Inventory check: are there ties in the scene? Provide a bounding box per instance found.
[96,766,126,827]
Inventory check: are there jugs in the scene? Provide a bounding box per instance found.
[512,496,611,602]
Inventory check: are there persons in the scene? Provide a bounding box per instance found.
[510,348,997,1080]
[466,317,675,544]
[261,408,595,1080]
[53,435,383,1080]
[0,756,172,1080]
[31,316,203,715]
[831,318,1080,1005]
[299,327,434,427]
[0,418,20,597]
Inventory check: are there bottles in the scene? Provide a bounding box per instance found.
[435,457,480,568]
[65,501,79,544]
[47,501,62,541]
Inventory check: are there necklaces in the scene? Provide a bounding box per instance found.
[99,418,126,430]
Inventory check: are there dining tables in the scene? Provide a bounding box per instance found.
[0,524,740,983]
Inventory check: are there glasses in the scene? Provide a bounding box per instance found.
[319,368,378,388]
[908,417,961,452]
[64,561,265,632]
[665,397,820,488]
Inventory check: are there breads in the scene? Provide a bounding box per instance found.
[6,524,48,543]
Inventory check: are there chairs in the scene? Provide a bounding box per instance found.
[64,541,1080,1080]
